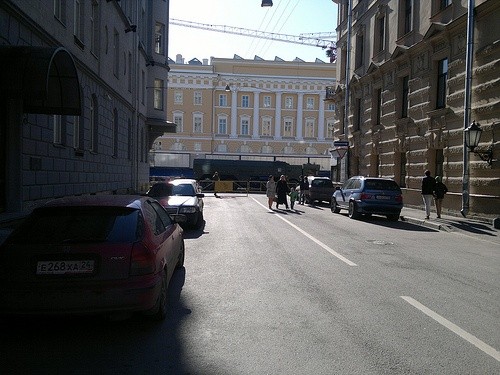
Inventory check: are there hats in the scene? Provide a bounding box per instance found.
[435,176,441,182]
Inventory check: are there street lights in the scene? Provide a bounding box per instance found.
[210,84,230,152]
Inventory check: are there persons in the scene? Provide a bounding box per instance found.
[433,176,448,219]
[213,171,220,197]
[275,175,292,209]
[266,175,276,209]
[298,175,304,205]
[301,176,309,205]
[421,170,436,219]
[287,187,298,210]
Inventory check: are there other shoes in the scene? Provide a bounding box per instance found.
[425,217,430,219]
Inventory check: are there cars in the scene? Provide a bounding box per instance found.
[147,181,204,229]
[0,195,185,329]
[174,178,203,195]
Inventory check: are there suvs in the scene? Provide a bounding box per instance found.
[295,175,335,205]
[331,176,404,221]
